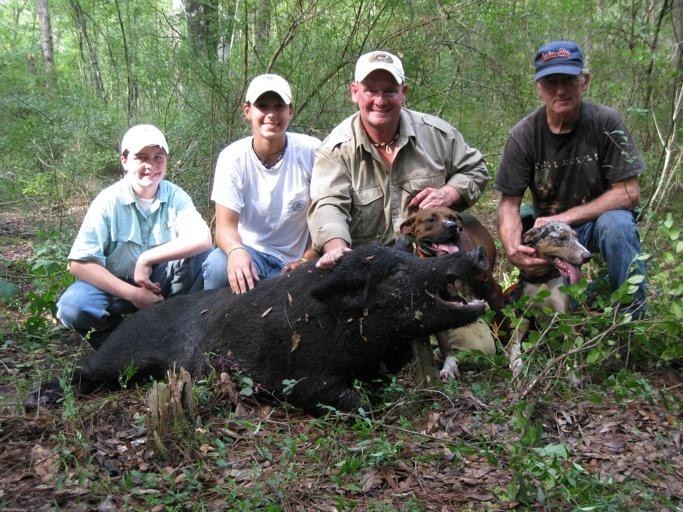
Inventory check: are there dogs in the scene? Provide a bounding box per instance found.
[394,203,499,382]
[502,221,595,372]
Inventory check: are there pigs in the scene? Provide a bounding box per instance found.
[21,242,490,423]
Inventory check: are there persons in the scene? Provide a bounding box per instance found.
[56,123,214,352]
[199,73,322,299]
[306,51,492,272]
[496,39,649,320]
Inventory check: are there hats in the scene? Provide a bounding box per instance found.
[533,41,583,81]
[246,74,292,105]
[354,51,404,85]
[121,124,169,155]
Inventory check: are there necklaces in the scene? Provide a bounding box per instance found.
[369,133,401,156]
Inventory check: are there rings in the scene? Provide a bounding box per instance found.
[284,264,289,271]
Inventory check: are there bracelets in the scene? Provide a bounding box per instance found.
[226,245,247,257]
[298,256,313,266]
